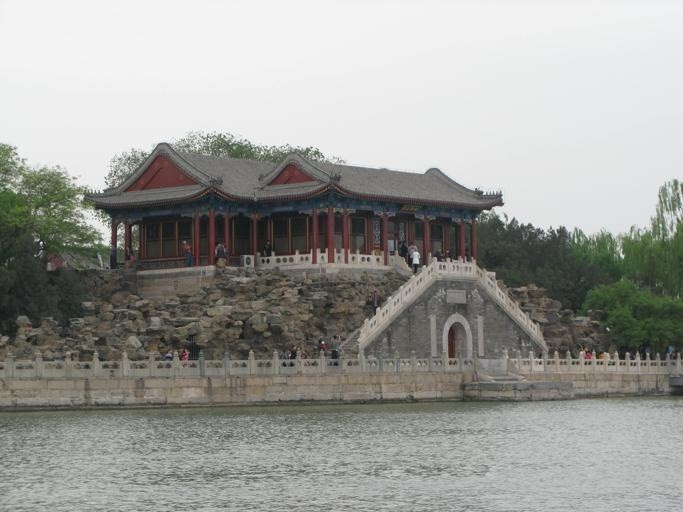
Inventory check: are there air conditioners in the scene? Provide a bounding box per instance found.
[239,253,255,268]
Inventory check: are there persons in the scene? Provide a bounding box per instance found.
[180,237,188,258]
[184,244,193,269]
[264,240,270,258]
[577,346,682,364]
[110,242,117,269]
[213,240,227,260]
[387,238,470,273]
[284,345,309,366]
[164,349,171,366]
[372,289,381,314]
[179,347,191,369]
[125,245,134,259]
[317,337,325,350]
[330,335,341,365]
